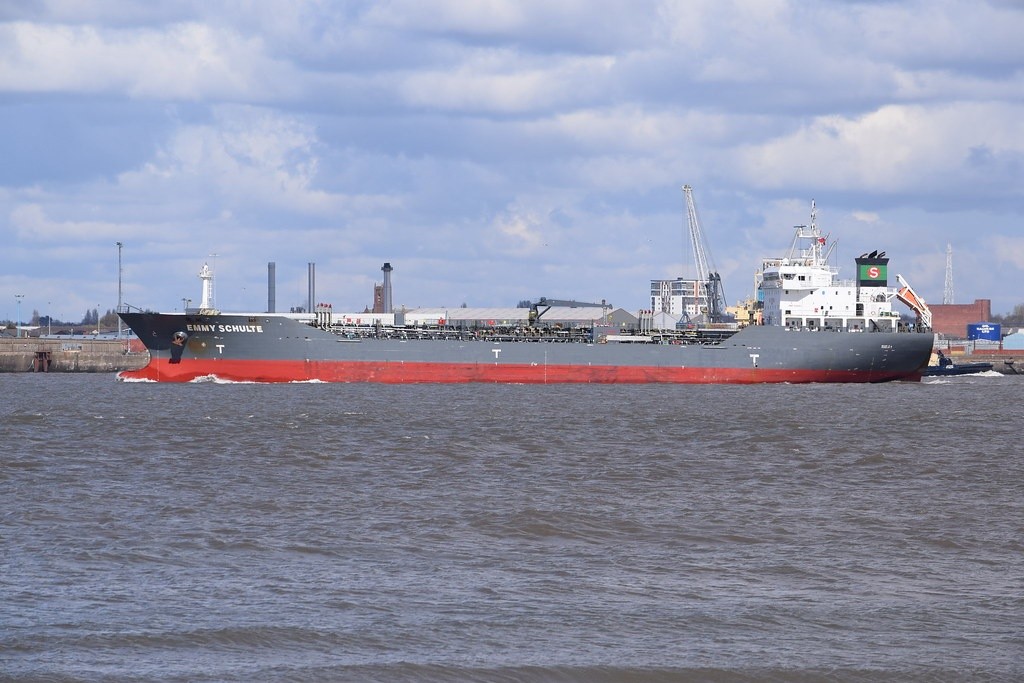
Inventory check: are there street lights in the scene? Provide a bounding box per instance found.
[14,294,25,337]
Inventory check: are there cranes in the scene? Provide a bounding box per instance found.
[676,183,740,334]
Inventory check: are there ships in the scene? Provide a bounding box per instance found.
[113,199,937,386]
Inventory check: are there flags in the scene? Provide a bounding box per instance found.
[818,238,825,245]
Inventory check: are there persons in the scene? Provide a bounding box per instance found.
[937,350,946,366]
[422,321,428,330]
